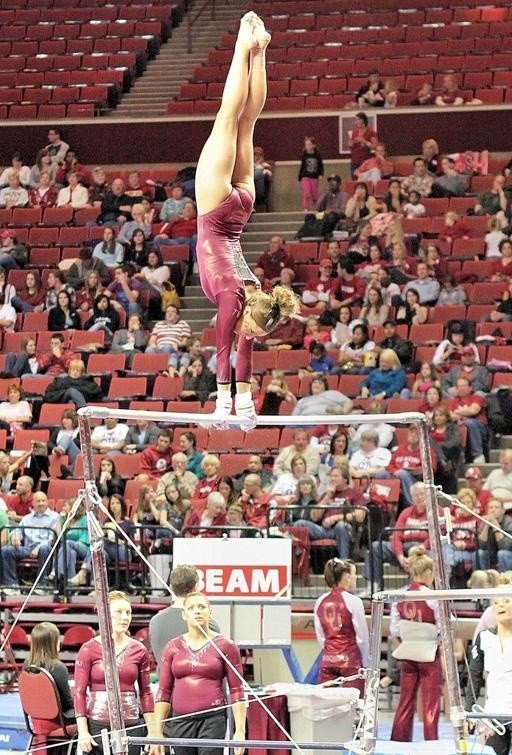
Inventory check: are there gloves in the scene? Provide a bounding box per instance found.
[211,390,233,431]
[235,391,260,433]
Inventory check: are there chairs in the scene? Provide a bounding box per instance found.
[0,1,512,755]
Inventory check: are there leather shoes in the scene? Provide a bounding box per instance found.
[68,575,87,584]
[162,371,169,377]
[174,371,179,378]
[61,464,74,477]
[44,574,55,583]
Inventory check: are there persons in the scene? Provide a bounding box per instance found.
[313,558,370,746]
[463,586,511,755]
[192,7,274,434]
[22,565,249,755]
[0,68,512,599]
[390,546,451,742]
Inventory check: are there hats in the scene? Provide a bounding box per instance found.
[465,467,483,479]
[383,319,397,326]
[327,174,341,182]
[460,346,474,355]
[320,259,332,267]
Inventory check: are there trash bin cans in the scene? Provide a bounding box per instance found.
[287,686,360,755]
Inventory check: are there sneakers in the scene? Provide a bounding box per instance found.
[3,588,21,595]
[55,448,65,456]
[379,675,394,689]
[358,587,382,601]
